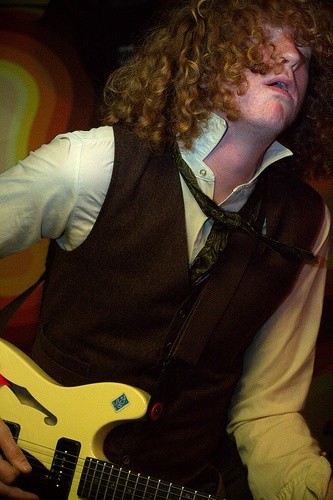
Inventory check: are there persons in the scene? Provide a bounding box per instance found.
[0,0,333,500]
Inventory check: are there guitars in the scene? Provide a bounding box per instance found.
[0,338,227,500]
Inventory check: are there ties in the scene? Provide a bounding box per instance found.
[156,142,316,297]
